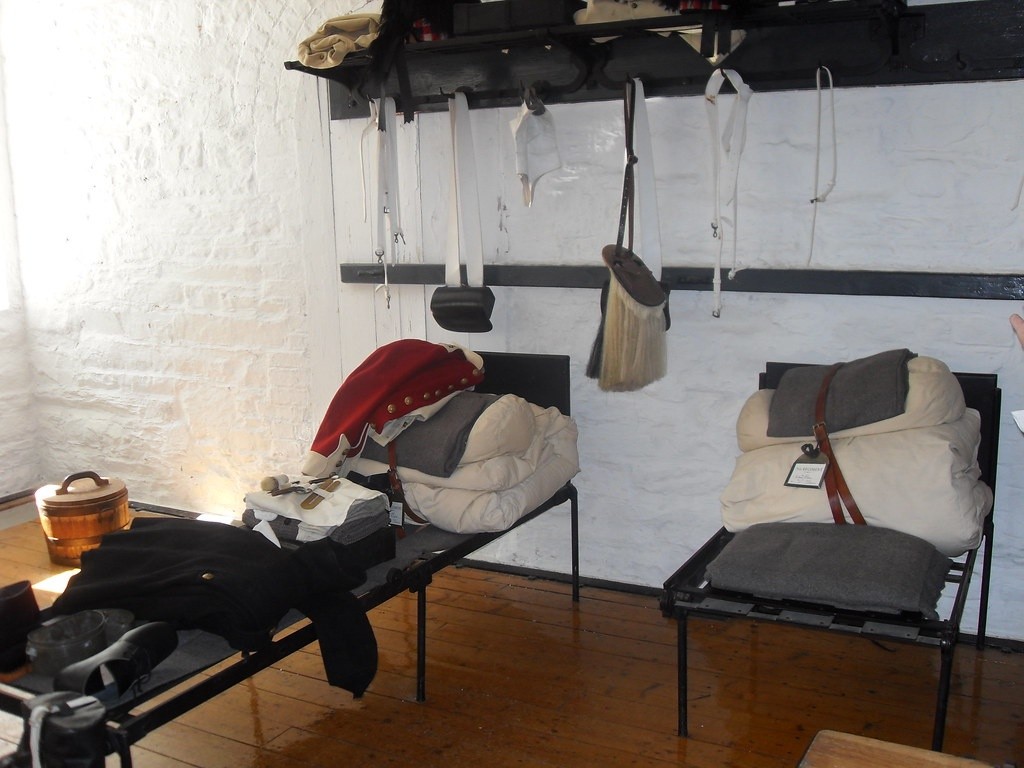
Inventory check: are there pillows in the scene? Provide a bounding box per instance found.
[459,392,535,465]
[737,356,967,452]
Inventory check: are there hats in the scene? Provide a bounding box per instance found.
[0,581,55,671]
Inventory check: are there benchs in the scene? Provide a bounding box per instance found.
[0,349,580,768]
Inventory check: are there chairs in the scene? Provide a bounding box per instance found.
[661,361,1005,750]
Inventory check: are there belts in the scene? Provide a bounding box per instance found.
[26,611,110,674]
[812,362,866,525]
[388,441,428,524]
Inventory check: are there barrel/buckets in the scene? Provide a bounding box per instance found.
[34,471,132,569]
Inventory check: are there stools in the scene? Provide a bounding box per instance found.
[796,729,1019,768]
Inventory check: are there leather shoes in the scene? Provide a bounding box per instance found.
[54,621,179,696]
